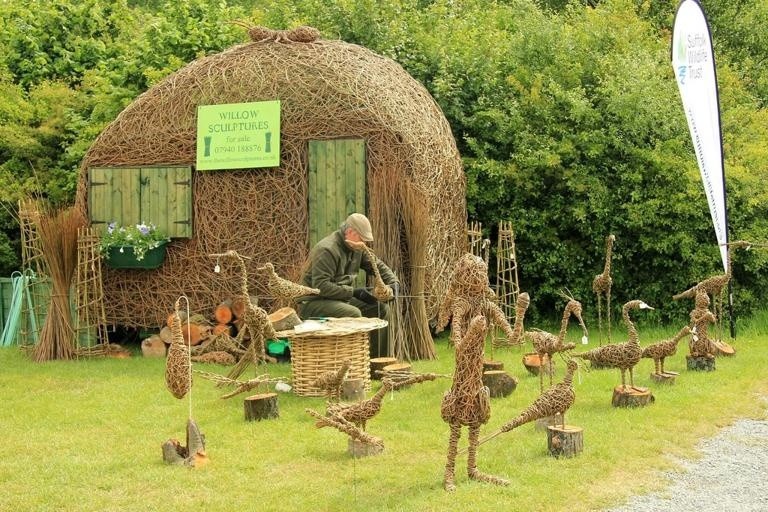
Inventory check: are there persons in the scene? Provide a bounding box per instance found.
[295,210,399,380]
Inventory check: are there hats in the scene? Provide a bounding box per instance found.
[347,212,374,243]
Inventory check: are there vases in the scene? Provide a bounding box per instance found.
[103,239,167,270]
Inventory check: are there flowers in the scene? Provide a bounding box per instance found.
[100,221,171,261]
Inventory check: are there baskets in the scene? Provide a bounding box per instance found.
[288,332,372,398]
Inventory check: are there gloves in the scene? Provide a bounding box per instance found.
[353,286,378,306]
